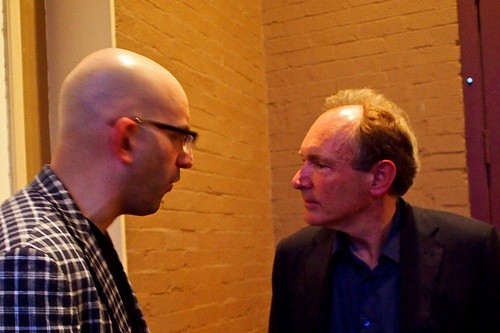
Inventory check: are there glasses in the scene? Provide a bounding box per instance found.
[107,115,198,157]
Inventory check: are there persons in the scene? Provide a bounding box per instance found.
[267,86,500,333]
[0,46,200,333]
